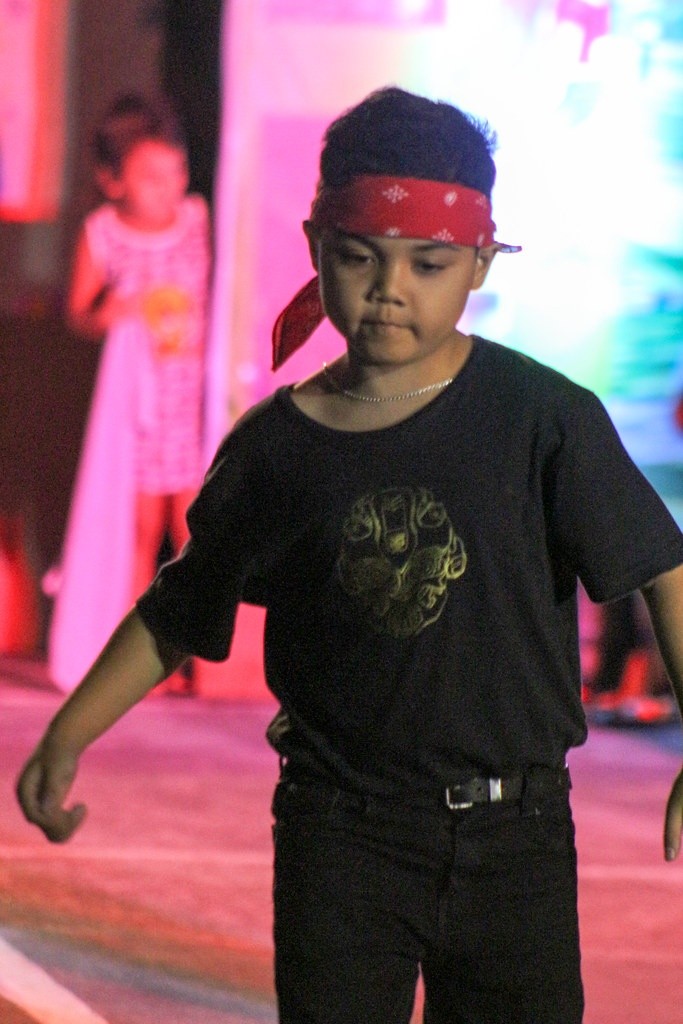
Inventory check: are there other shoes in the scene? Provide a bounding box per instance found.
[591,701,640,726]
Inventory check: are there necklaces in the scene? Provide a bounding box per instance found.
[322,361,454,403]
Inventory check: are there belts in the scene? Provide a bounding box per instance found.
[444,768,570,809]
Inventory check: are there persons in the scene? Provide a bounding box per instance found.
[16,86,683,1024]
[45,97,215,694]
